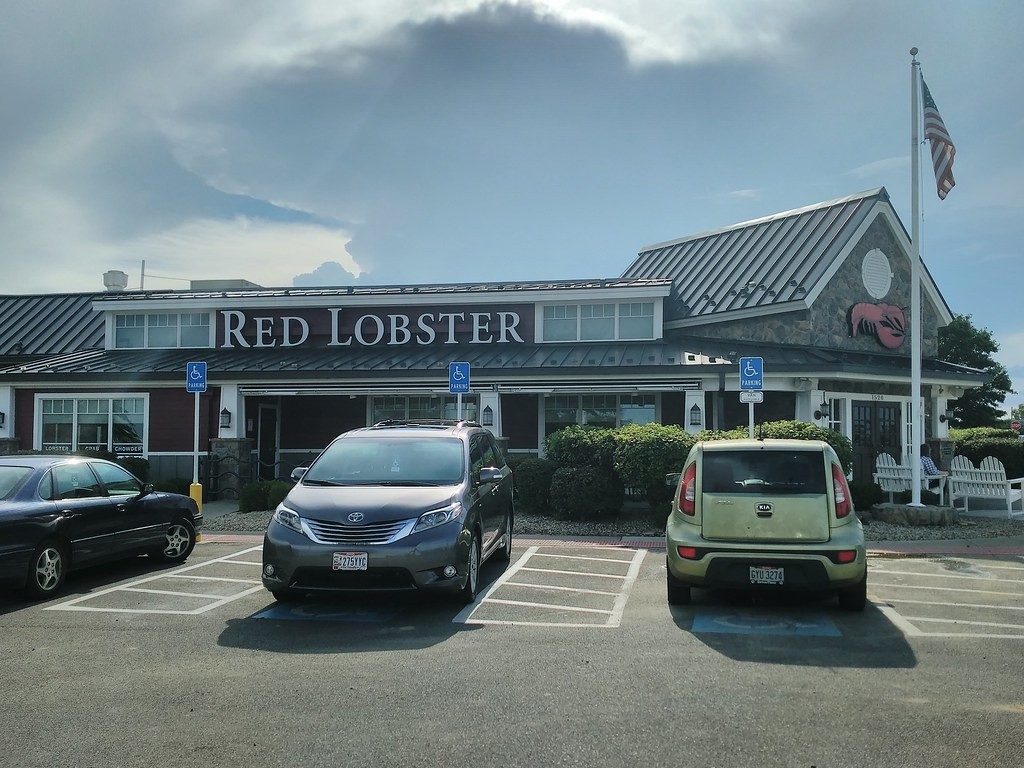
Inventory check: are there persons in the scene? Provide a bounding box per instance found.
[920,444,950,498]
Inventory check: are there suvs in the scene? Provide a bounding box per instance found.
[261,420,516,607]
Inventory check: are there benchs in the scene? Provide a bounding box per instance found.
[874,453,947,504]
[948,455,1024,517]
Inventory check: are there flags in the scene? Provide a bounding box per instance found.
[919,64,957,201]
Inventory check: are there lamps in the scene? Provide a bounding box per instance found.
[814,400,831,420]
[690,403,701,425]
[940,407,954,423]
[0,412,4,428]
[482,405,493,426]
[220,408,231,428]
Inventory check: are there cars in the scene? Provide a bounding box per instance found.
[0,454,203,603]
[662,435,872,613]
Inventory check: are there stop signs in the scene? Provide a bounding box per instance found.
[1011,421,1021,431]
